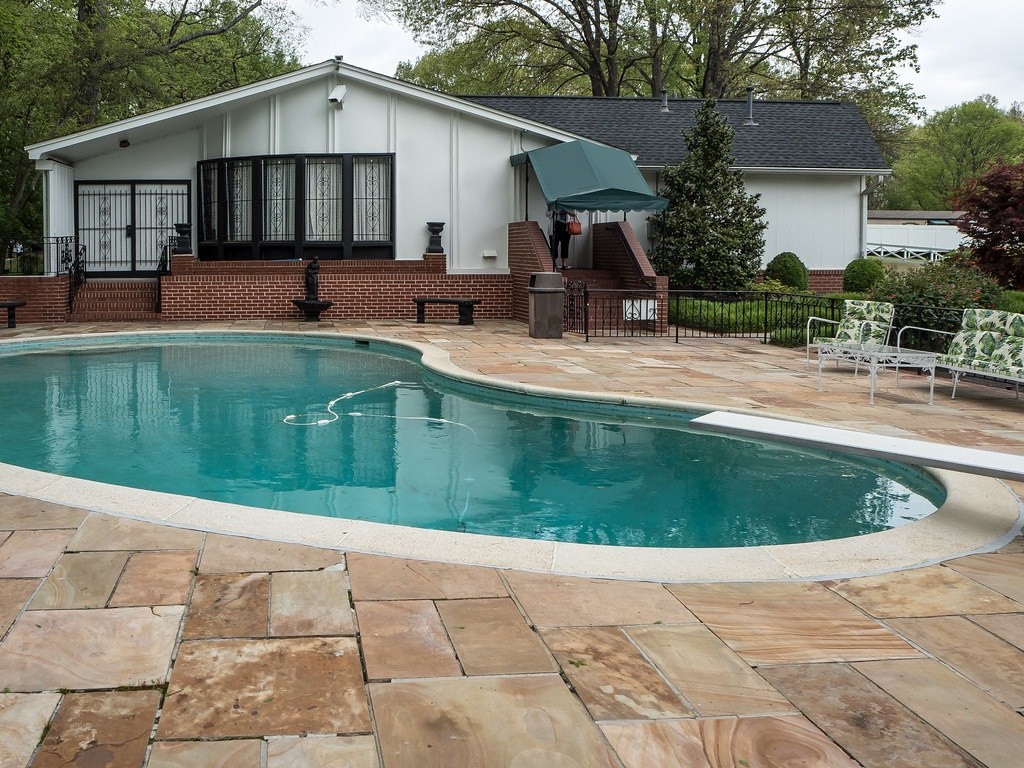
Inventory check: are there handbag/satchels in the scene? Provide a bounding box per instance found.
[567,214,583,236]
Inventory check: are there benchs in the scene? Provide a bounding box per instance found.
[412,297,482,325]
[0,301,26,328]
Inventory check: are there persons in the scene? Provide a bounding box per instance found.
[545,206,577,270]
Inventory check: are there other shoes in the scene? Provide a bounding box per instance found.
[562,263,576,270]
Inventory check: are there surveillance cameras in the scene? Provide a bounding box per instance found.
[327,84,346,108]
[335,54,343,62]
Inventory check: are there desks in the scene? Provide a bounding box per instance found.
[818,342,939,405]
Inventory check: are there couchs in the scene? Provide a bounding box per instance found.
[897,307,1024,401]
[807,300,897,377]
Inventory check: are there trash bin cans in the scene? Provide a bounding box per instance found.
[527,270,565,339]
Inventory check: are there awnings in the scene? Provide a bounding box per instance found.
[510,138,670,216]
